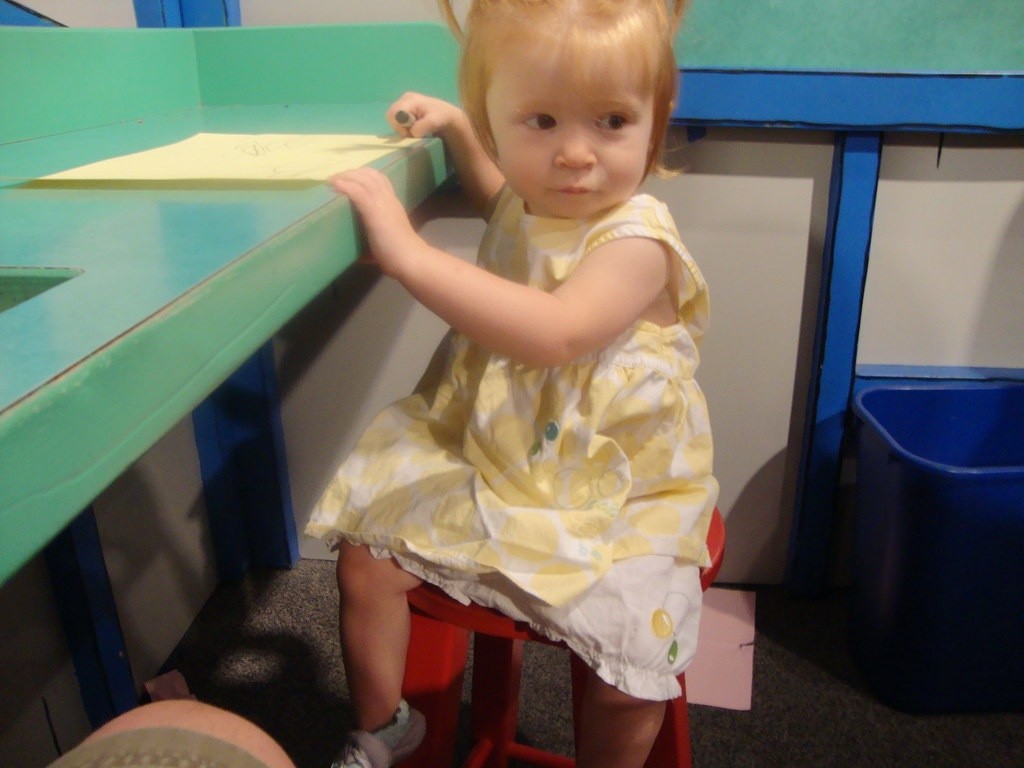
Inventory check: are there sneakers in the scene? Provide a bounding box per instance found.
[331,699,427,768]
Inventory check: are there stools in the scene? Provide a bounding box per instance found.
[408,502,727,768]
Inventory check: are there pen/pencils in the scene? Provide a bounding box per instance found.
[395,110,415,128]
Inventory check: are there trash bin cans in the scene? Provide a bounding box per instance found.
[852,379,1024,710]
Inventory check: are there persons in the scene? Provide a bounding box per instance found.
[42,697,294,768]
[304,0,717,768]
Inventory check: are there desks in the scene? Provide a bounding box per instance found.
[0,94,464,727]
